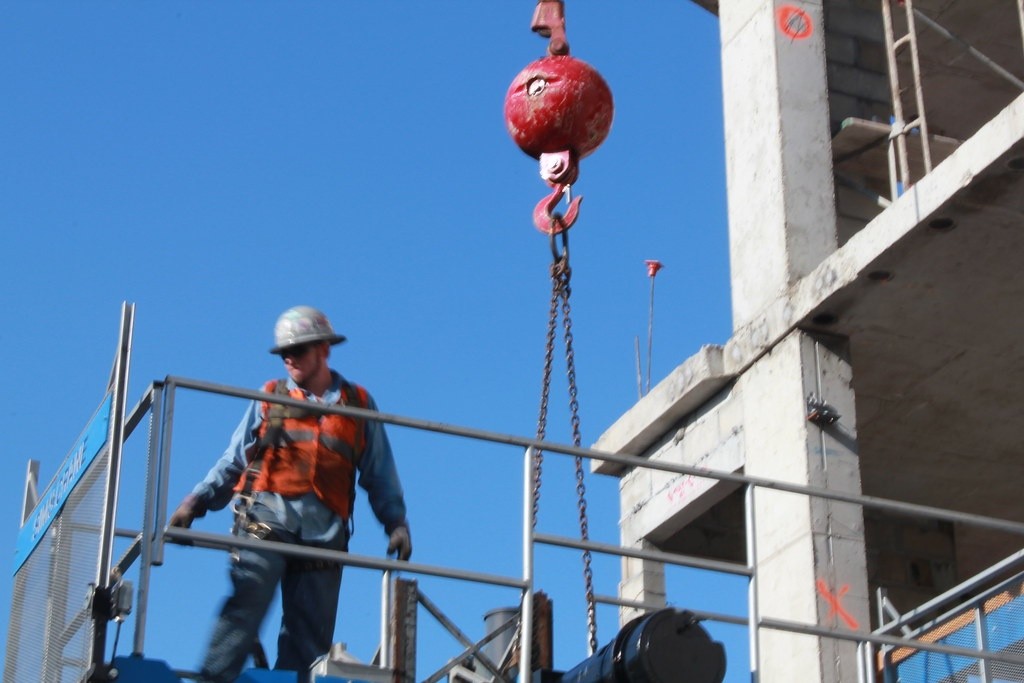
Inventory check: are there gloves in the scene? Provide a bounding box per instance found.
[386,525,411,562]
[170,502,196,528]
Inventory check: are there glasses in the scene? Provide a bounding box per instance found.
[279,347,305,360]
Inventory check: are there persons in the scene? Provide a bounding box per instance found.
[169,305,413,683]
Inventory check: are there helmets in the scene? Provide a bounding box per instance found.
[268,305,344,354]
